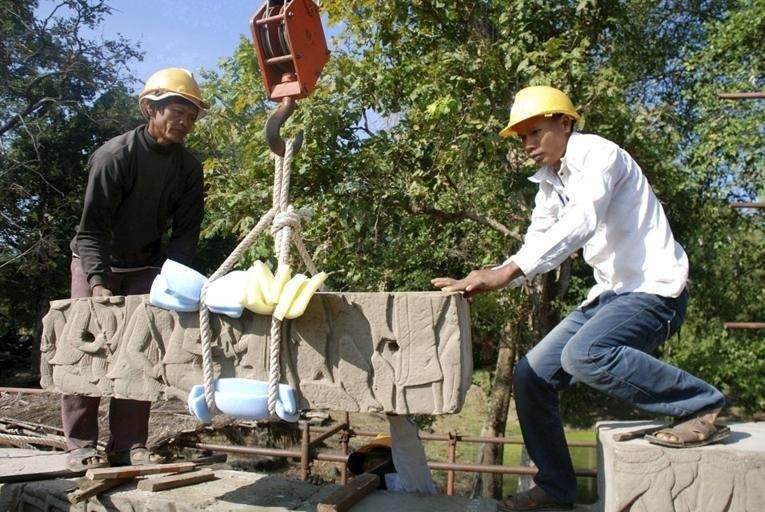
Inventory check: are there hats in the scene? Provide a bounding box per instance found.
[498,85,581,136]
[139,92,207,123]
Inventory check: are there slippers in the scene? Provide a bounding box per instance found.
[108,450,165,466]
[64,447,111,472]
[496,486,573,511]
[645,416,731,448]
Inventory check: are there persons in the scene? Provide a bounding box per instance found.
[60,67,212,472]
[430,86,732,512]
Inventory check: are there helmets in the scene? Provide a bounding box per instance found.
[139,68,210,109]
